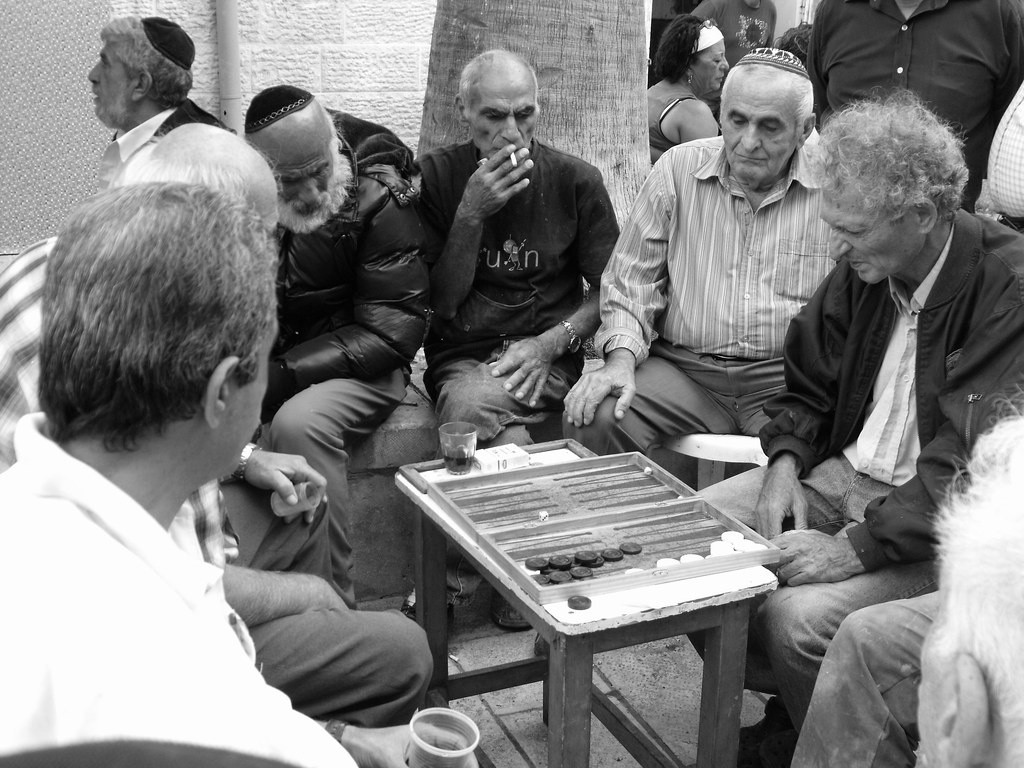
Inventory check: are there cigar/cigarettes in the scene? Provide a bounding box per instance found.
[510,152,517,166]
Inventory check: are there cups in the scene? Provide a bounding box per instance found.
[439,422,478,474]
[270,481,320,517]
[408,707,480,768]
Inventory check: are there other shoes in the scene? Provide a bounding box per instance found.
[489,585,534,631]
[738,709,798,768]
[399,586,456,626]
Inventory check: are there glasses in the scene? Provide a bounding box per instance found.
[696,18,717,29]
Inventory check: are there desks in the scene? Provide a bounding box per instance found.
[396,438,776,768]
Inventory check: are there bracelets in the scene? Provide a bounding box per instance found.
[326,717,351,744]
[233,442,264,481]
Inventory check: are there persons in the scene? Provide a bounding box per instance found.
[685,85,1024,768]
[563,46,837,461]
[647,13,729,168]
[89,16,237,194]
[807,0,1024,178]
[401,49,622,632]
[987,79,1024,233]
[0,180,484,768]
[690,0,777,126]
[0,123,433,730]
[913,380,1024,768]
[772,19,813,70]
[245,85,430,610]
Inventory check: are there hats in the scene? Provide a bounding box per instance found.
[141,17,196,71]
[245,86,314,133]
[734,47,809,78]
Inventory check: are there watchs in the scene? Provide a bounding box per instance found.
[559,320,581,353]
